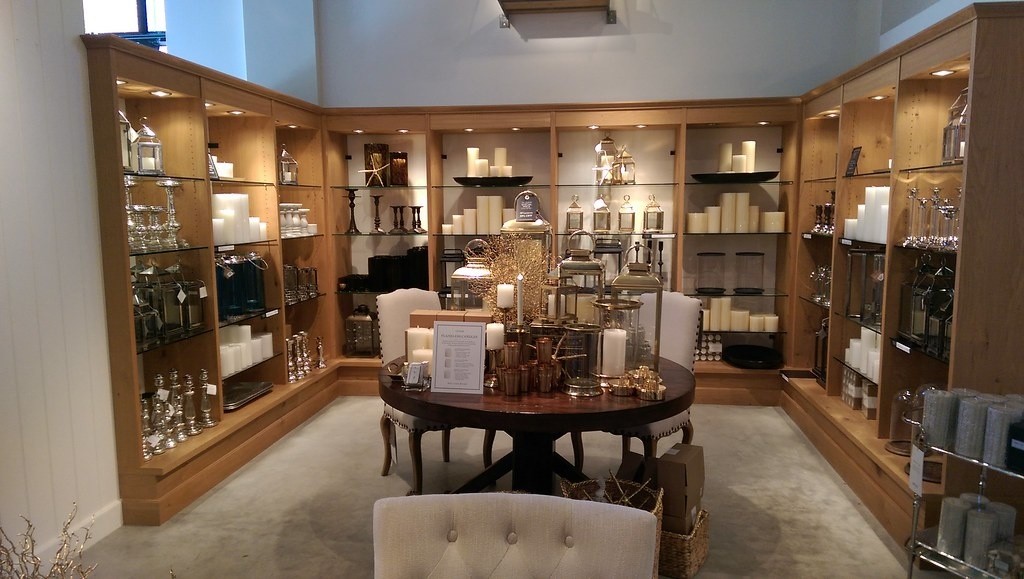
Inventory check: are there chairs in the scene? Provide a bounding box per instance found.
[572,290,704,479]
[376,288,496,497]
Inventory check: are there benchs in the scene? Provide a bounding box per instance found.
[372,492,657,579]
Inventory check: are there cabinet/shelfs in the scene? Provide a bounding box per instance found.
[82,30,340,526]
[779,0,1024,571]
[900,406,1021,576]
[320,94,802,407]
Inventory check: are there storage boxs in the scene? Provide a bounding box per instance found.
[1006,422,1024,476]
[285,325,292,339]
[409,309,440,328]
[404,330,408,362]
[389,151,409,187]
[862,379,878,421]
[364,143,390,187]
[656,442,706,535]
[464,311,495,324]
[614,451,644,484]
[437,310,467,322]
[841,366,862,409]
[693,334,722,361]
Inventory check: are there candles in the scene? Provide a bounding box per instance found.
[601,155,614,179]
[841,326,881,420]
[623,172,630,180]
[945,323,951,337]
[716,139,756,172]
[921,387,1024,469]
[936,493,1016,570]
[497,282,514,308]
[212,193,267,245]
[843,185,890,244]
[517,273,524,324]
[929,316,939,337]
[407,325,434,378]
[219,325,274,377]
[142,156,155,170]
[685,192,786,234]
[487,322,505,350]
[704,298,780,332]
[466,147,513,177]
[959,141,965,158]
[548,293,566,316]
[285,171,291,182]
[694,334,723,361]
[602,329,627,375]
[912,311,925,333]
[212,155,234,178]
[441,195,515,233]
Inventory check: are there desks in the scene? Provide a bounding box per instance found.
[376,350,697,497]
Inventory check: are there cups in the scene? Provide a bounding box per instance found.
[496,360,561,395]
[565,323,604,397]
[504,342,520,368]
[536,338,554,364]
